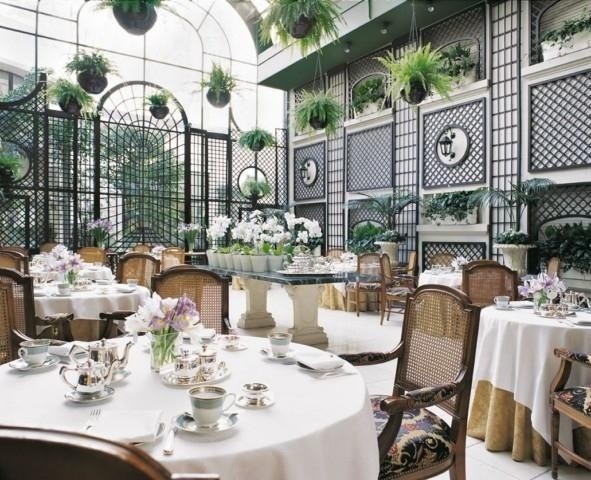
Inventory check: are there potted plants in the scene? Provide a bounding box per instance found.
[224,247,233,269]
[540,29,561,60]
[250,246,267,272]
[268,244,284,271]
[48,79,92,113]
[549,222,591,278]
[436,43,474,90]
[423,191,476,225]
[239,129,273,152]
[244,181,269,207]
[349,189,422,264]
[469,178,551,276]
[353,79,385,113]
[66,51,119,92]
[218,248,227,268]
[210,248,219,266]
[0,150,20,182]
[293,90,343,134]
[206,248,214,264]
[259,0,338,56]
[199,64,236,109]
[231,244,241,271]
[375,45,457,106]
[240,247,252,271]
[94,0,161,36]
[147,92,169,118]
[349,223,381,257]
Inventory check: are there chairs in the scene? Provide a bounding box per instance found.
[116,253,160,296]
[97,269,230,340]
[0,267,71,365]
[347,252,383,316]
[328,250,343,258]
[379,254,416,325]
[548,256,559,277]
[0,249,29,277]
[431,254,458,270]
[336,285,480,480]
[40,243,59,257]
[133,246,151,254]
[549,345,591,480]
[1,426,219,480]
[460,265,517,317]
[391,251,416,280]
[4,248,28,259]
[157,247,184,275]
[79,247,105,267]
[462,261,500,285]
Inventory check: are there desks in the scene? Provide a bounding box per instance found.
[197,265,348,345]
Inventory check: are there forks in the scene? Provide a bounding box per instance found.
[81,409,103,428]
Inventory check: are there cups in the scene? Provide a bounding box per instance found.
[56,283,71,297]
[244,383,269,404]
[494,296,510,308]
[189,387,236,422]
[541,304,571,317]
[124,281,138,292]
[434,262,444,275]
[177,348,200,378]
[270,334,291,356]
[18,340,51,365]
[200,345,216,373]
[230,329,241,347]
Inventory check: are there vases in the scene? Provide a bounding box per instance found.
[147,332,178,373]
[534,292,552,315]
[97,242,105,251]
[312,245,321,256]
[456,264,463,270]
[55,272,70,284]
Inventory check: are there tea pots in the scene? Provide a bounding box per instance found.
[561,290,588,314]
[60,358,118,393]
[70,339,135,371]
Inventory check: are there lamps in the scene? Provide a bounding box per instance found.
[300,158,311,181]
[439,125,456,160]
[343,42,351,53]
[380,23,390,35]
[426,1,437,14]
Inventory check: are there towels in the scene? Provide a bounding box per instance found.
[184,322,214,340]
[570,310,589,323]
[87,407,163,440]
[288,347,343,371]
[48,339,97,356]
[510,297,535,308]
[112,283,136,293]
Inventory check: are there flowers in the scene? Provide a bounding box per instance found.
[239,226,254,255]
[296,230,308,245]
[38,245,84,280]
[267,232,290,256]
[249,234,265,257]
[179,224,200,250]
[231,225,244,255]
[125,295,200,364]
[307,222,323,246]
[207,214,228,242]
[518,272,566,301]
[89,219,114,244]
[452,256,468,269]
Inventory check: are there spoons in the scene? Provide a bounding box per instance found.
[225,318,234,331]
[162,415,177,454]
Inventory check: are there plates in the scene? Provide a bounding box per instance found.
[13,357,56,372]
[300,355,345,372]
[224,342,248,353]
[126,422,167,446]
[239,396,274,408]
[176,413,238,434]
[68,387,115,403]
[159,361,230,384]
